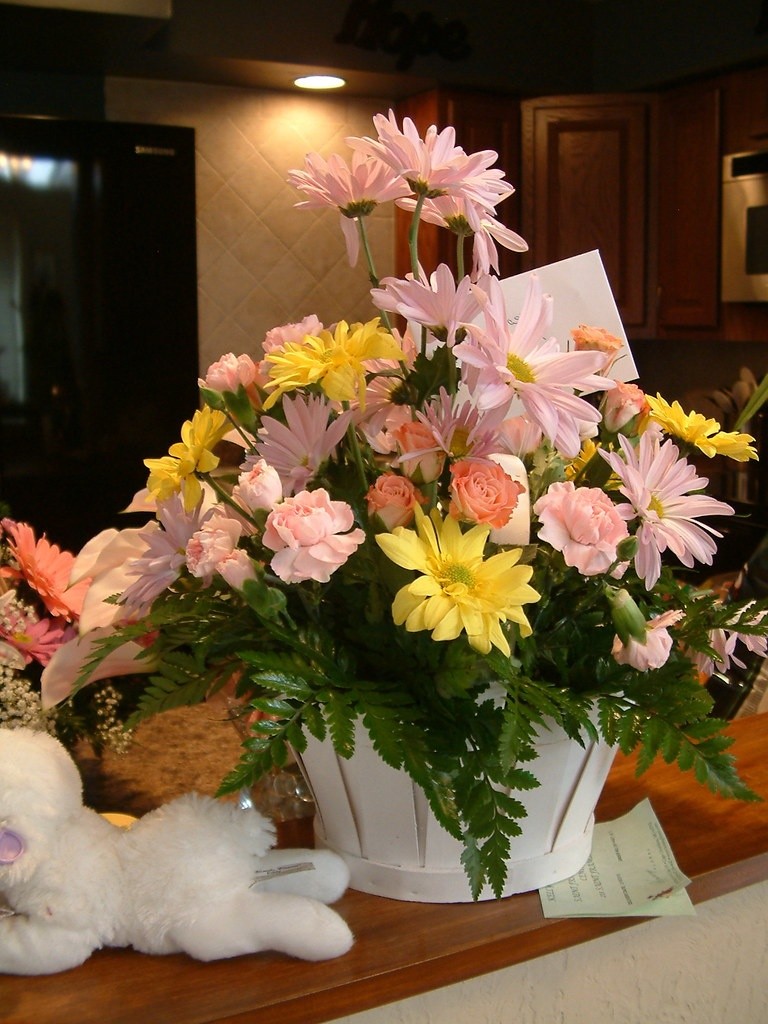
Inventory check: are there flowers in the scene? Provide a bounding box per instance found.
[0,109,768,696]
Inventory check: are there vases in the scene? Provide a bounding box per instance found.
[273,684,642,905]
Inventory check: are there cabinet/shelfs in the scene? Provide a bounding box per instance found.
[522,61,768,344]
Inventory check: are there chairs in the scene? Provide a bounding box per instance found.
[38,672,206,767]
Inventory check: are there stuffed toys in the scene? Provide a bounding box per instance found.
[0,725,355,975]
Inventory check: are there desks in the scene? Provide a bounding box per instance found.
[0,711,768,1024]
